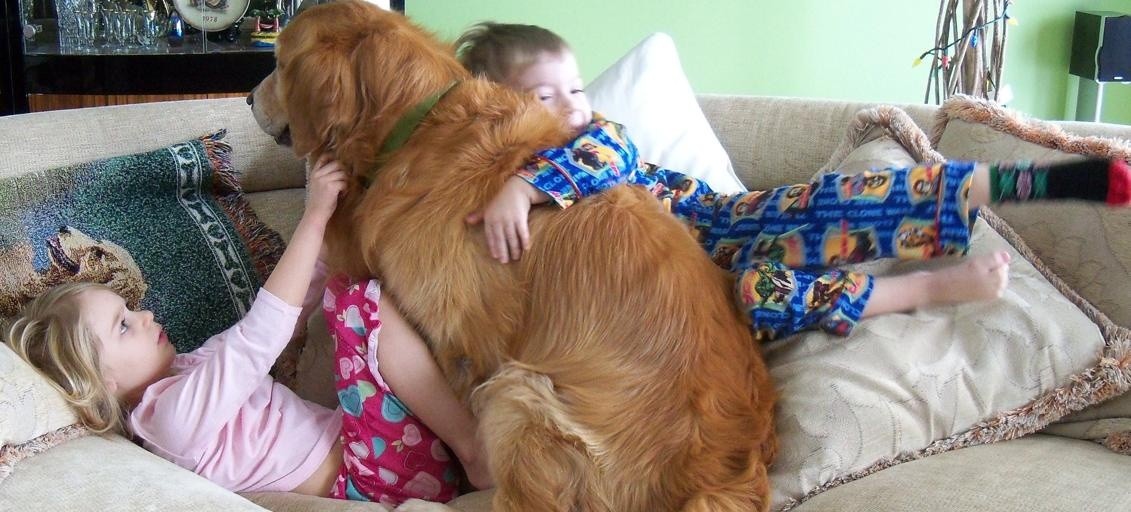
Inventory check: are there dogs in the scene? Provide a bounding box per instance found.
[0,222,149,330]
[246,0,786,512]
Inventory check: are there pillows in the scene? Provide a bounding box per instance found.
[1,339,97,483]
[584,29,746,197]
[930,94,1130,458]
[763,103,1131,512]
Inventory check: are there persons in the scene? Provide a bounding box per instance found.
[4,152,497,509]
[450,19,1131,341]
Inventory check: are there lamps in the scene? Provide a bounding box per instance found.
[1069,9,1131,121]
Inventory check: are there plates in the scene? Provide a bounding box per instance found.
[175,0,251,36]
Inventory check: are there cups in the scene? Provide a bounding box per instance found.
[54,0,164,52]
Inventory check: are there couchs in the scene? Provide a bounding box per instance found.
[1,94,1131,512]
